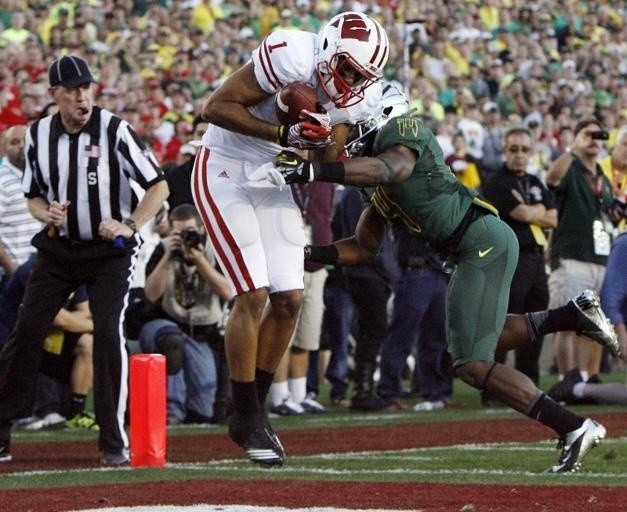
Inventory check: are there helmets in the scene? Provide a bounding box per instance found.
[315,12,390,107]
[343,81,409,156]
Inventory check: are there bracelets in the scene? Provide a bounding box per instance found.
[304,244,338,265]
[276,123,288,145]
[314,160,345,186]
[124,218,138,234]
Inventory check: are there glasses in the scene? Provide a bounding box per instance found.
[510,145,530,153]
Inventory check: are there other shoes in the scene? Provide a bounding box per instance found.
[328,386,467,412]
[2,444,10,462]
[16,411,65,431]
[102,449,130,465]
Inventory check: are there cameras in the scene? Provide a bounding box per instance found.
[181,228,206,247]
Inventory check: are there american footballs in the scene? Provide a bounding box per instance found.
[275,83,319,125]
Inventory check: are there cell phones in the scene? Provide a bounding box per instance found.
[591,131,609,140]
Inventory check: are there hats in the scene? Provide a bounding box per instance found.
[50,57,100,90]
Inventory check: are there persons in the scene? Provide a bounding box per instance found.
[0,1,627,407]
[1,55,170,462]
[187,10,389,467]
[283,76,607,474]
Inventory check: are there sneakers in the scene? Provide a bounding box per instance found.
[226,412,285,468]
[302,398,327,413]
[571,291,621,360]
[547,370,602,405]
[545,418,606,474]
[65,410,99,432]
[271,397,306,414]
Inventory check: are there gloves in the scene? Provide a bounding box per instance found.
[273,103,334,184]
